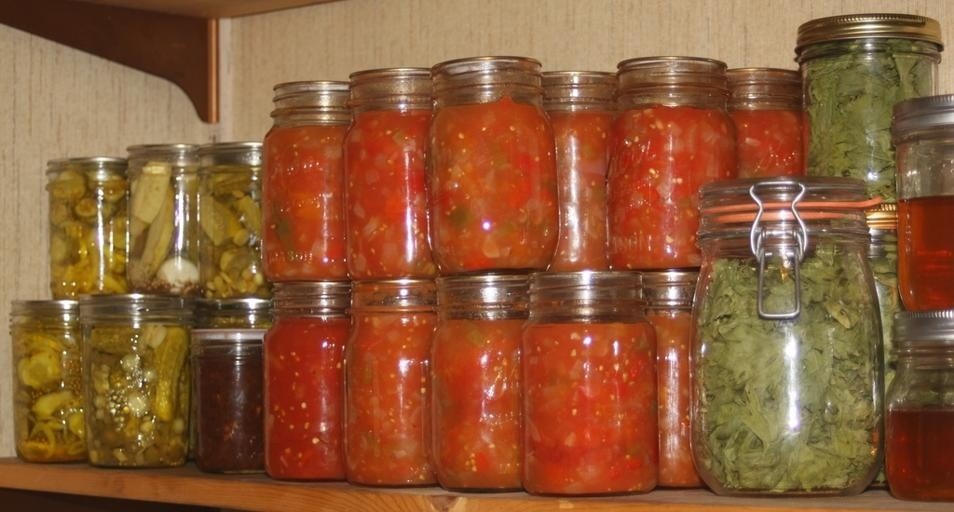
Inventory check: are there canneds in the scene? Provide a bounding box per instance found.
[8,12,954,504]
